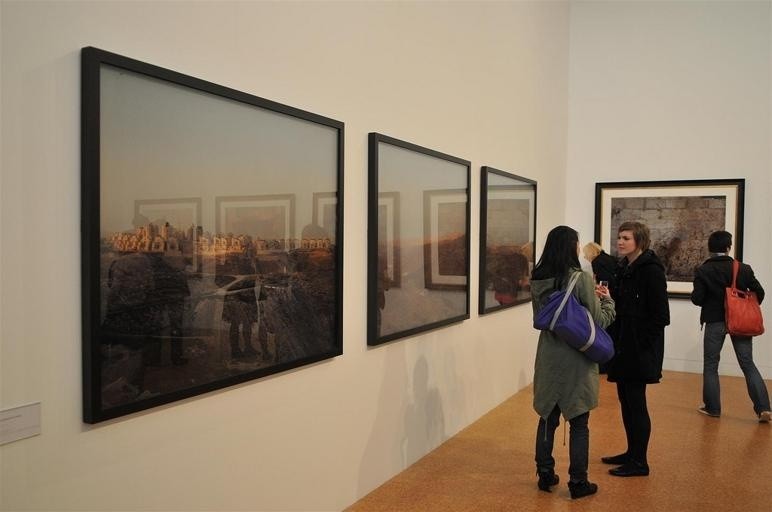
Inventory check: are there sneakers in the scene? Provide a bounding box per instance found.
[539,474,559,489]
[567,482,597,499]
[698,406,721,416]
[759,410,770,423]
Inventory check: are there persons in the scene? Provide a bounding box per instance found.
[691,231,772,422]
[529,225,617,500]
[107,224,335,394]
[492,242,534,306]
[584,242,618,287]
[602,221,670,477]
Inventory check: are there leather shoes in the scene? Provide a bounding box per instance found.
[601,453,631,464]
[608,466,649,476]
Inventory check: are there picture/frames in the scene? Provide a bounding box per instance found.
[368,131,471,349]
[479,165,537,319]
[487,183,533,291]
[136,196,203,283]
[79,43,344,427]
[313,192,337,247]
[423,186,470,290]
[215,193,295,288]
[376,191,400,288]
[595,178,747,300]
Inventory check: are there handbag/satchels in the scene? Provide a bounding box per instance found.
[725,259,765,336]
[534,271,616,363]
